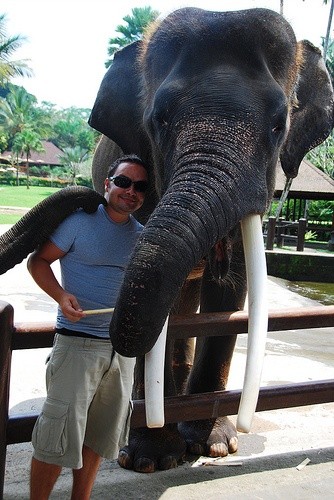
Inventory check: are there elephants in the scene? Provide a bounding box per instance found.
[0,185,108,277]
[87,6,334,473]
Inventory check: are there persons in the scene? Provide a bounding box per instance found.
[27,152,151,500]
